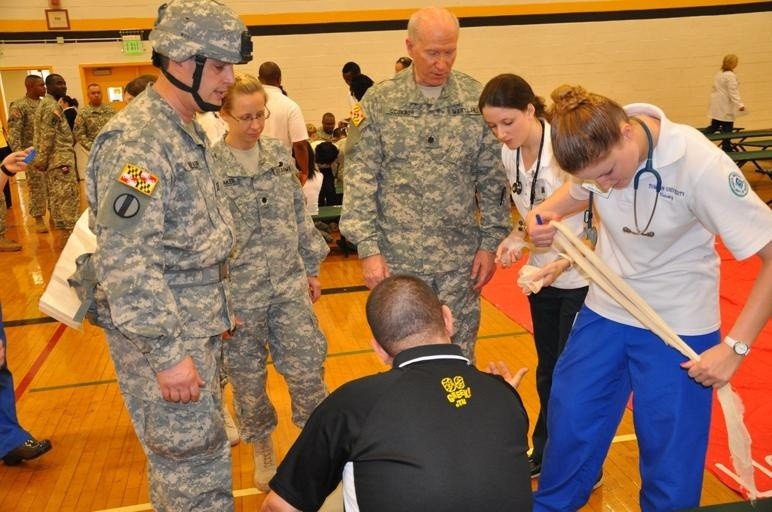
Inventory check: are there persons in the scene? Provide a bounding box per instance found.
[0,120,13,209]
[292,141,324,215]
[124,74,157,104]
[701,55,745,152]
[262,275,528,511]
[73,83,116,151]
[33,74,80,250]
[314,142,339,165]
[7,75,51,232]
[395,56,414,73]
[316,113,336,140]
[350,74,374,104]
[305,123,325,152]
[0,300,52,466]
[67,0,254,512]
[330,127,347,184]
[339,7,513,364]
[255,61,308,187]
[212,72,330,491]
[0,192,23,251]
[478,73,603,491]
[342,63,360,85]
[527,84,772,512]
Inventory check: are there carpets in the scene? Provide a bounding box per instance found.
[480,233,772,501]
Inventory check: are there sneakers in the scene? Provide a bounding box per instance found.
[528,455,541,478]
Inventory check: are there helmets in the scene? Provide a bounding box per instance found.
[148,0,253,66]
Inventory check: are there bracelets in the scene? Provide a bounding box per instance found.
[1,165,16,177]
[553,253,574,271]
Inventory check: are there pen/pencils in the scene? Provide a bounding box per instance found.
[535,213,542,225]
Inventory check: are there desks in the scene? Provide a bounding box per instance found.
[728,150,772,205]
[310,206,358,258]
[704,128,772,152]
[732,140,772,152]
[696,127,745,134]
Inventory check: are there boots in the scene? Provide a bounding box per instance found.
[221,405,238,445]
[34,216,49,233]
[252,437,279,491]
[0,235,22,251]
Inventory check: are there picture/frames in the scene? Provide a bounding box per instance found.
[45,9,71,30]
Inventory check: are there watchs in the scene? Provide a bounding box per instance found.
[723,336,750,356]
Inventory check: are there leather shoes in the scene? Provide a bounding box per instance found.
[3,436,51,465]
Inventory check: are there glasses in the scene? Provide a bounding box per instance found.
[226,103,271,124]
